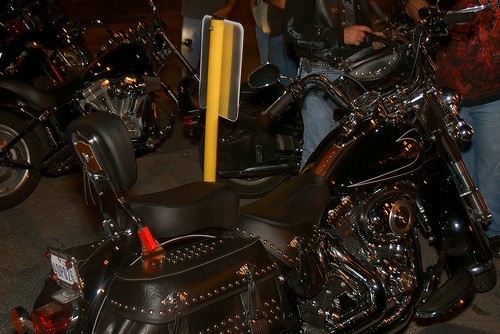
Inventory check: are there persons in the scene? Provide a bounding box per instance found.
[215,0,270,66]
[264,0,296,87]
[406,0,500,257]
[180,1,237,79]
[284,0,386,175]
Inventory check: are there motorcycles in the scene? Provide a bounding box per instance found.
[12,2,497,334]
[0,0,308,216]
